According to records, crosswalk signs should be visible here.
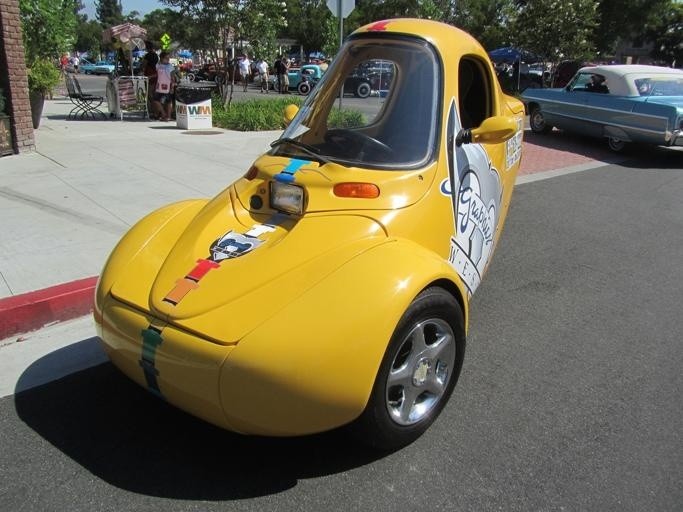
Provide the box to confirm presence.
[160,32,171,45]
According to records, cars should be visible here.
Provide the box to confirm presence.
[498,62,683,156]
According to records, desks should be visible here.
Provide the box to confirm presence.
[107,75,150,120]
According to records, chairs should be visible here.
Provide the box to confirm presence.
[64,74,108,120]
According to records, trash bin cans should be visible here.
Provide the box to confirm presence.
[175,85,213,129]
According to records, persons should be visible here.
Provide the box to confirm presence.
[60,40,291,122]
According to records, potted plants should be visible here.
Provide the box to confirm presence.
[0,86,15,156]
[26,55,61,129]
